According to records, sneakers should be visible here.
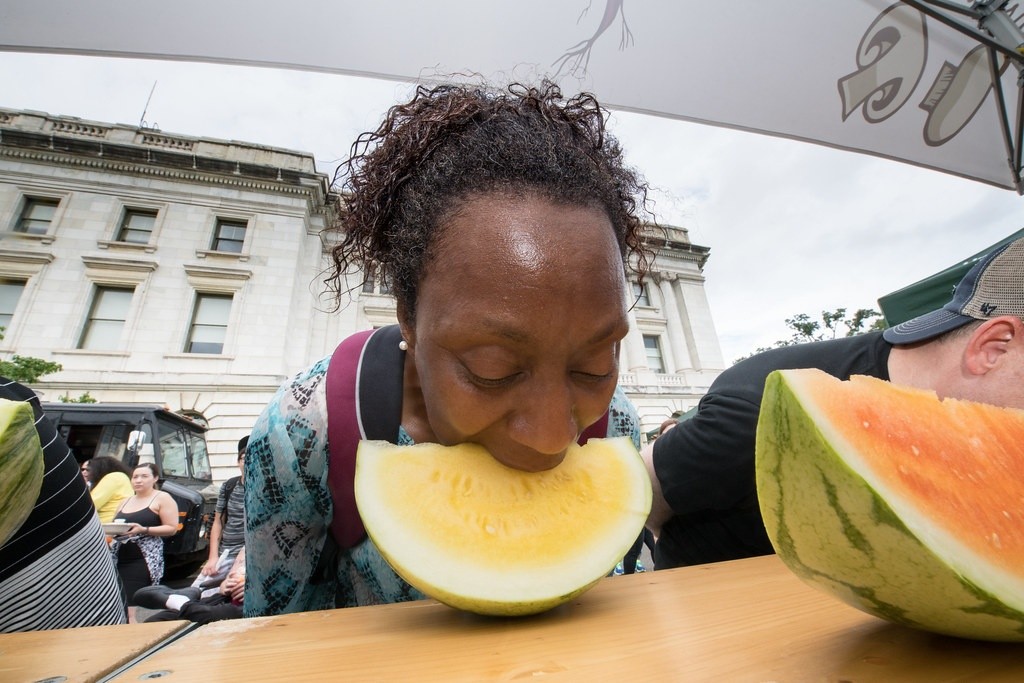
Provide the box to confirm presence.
[614,562,624,575]
[635,559,647,572]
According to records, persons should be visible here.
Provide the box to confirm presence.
[609,237,1024,575]
[0,376,250,635]
[244,71,666,617]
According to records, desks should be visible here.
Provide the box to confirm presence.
[0,553,1024,683]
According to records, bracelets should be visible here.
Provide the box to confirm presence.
[143,526,150,535]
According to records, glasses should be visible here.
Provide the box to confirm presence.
[81,468,88,471]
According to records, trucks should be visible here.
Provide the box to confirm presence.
[40,400,224,583]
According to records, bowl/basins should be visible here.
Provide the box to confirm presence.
[102,523,132,535]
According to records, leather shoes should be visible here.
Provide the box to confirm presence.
[133,585,201,609]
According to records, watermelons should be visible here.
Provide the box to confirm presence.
[353,435,654,614]
[0,397,43,547]
[755,367,1024,642]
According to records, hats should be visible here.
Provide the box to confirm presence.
[238,435,250,462]
[883,228,1024,345]
